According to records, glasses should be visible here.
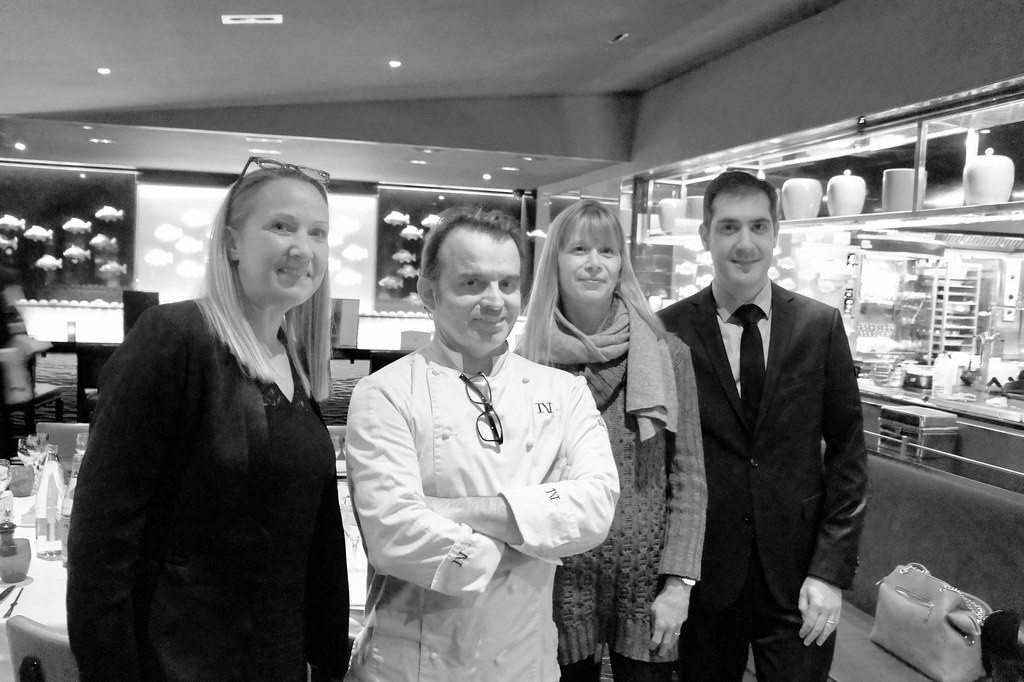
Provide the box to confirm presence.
[459,371,503,445]
[233,157,330,196]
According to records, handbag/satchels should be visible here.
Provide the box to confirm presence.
[869,563,992,682]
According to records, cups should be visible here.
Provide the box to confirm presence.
[7,465,35,497]
[0,459,12,491]
[0,538,31,583]
[0,492,14,523]
[16,433,48,465]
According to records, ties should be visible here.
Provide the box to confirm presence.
[734,304,766,402]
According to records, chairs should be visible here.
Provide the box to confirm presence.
[36,422,89,458]
[6,615,80,682]
[6,354,63,457]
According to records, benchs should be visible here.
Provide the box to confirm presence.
[745,448,1024,682]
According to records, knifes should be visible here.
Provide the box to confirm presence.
[0,586,15,600]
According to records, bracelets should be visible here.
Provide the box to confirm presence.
[678,577,697,586]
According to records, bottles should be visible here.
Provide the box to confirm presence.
[35,442,66,562]
[61,432,89,567]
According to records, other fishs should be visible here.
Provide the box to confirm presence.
[767,242,816,291]
[526,229,547,240]
[327,210,444,305]
[0,205,213,279]
[645,287,668,298]
[675,240,713,301]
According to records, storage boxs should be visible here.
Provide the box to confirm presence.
[400,331,431,350]
[878,404,957,459]
[331,298,360,350]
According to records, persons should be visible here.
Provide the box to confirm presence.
[655,171,870,682]
[342,208,621,681]
[68,170,350,681]
[514,199,707,682]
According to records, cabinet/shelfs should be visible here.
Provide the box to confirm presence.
[628,81,1024,431]
